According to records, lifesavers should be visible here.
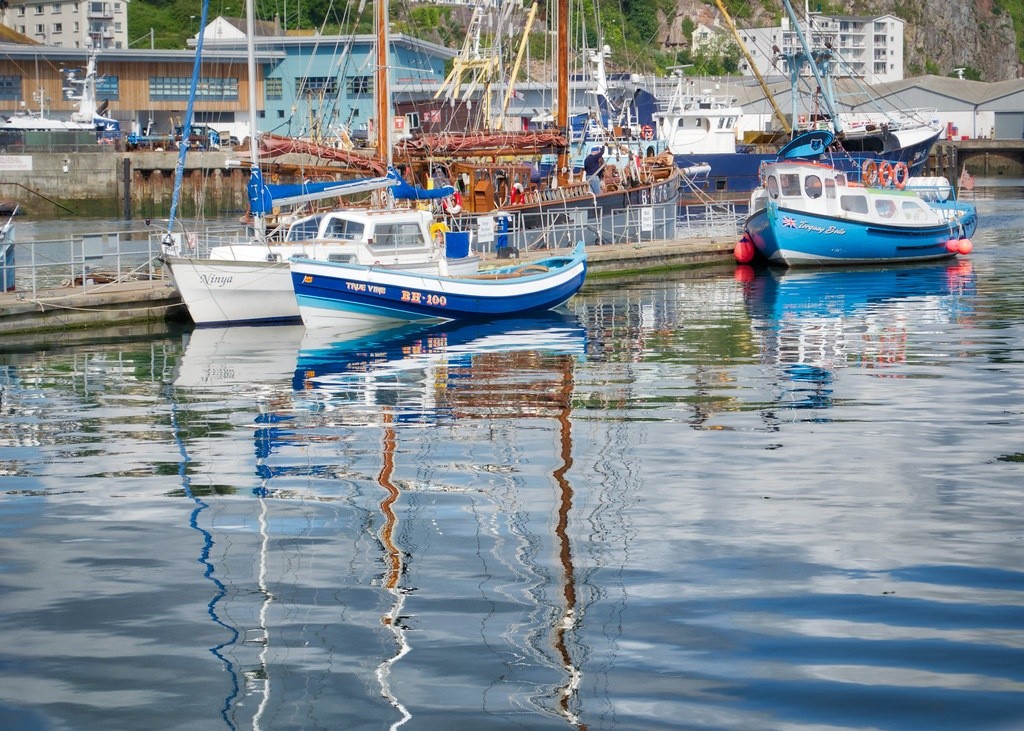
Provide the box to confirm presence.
[511,182,525,207]
[893,162,908,189]
[878,160,893,187]
[441,184,463,215]
[861,158,878,186]
[641,125,653,139]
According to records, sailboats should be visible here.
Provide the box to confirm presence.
[742,259,980,369]
[171,312,591,731]
[741,0,979,268]
[157,1,945,325]
